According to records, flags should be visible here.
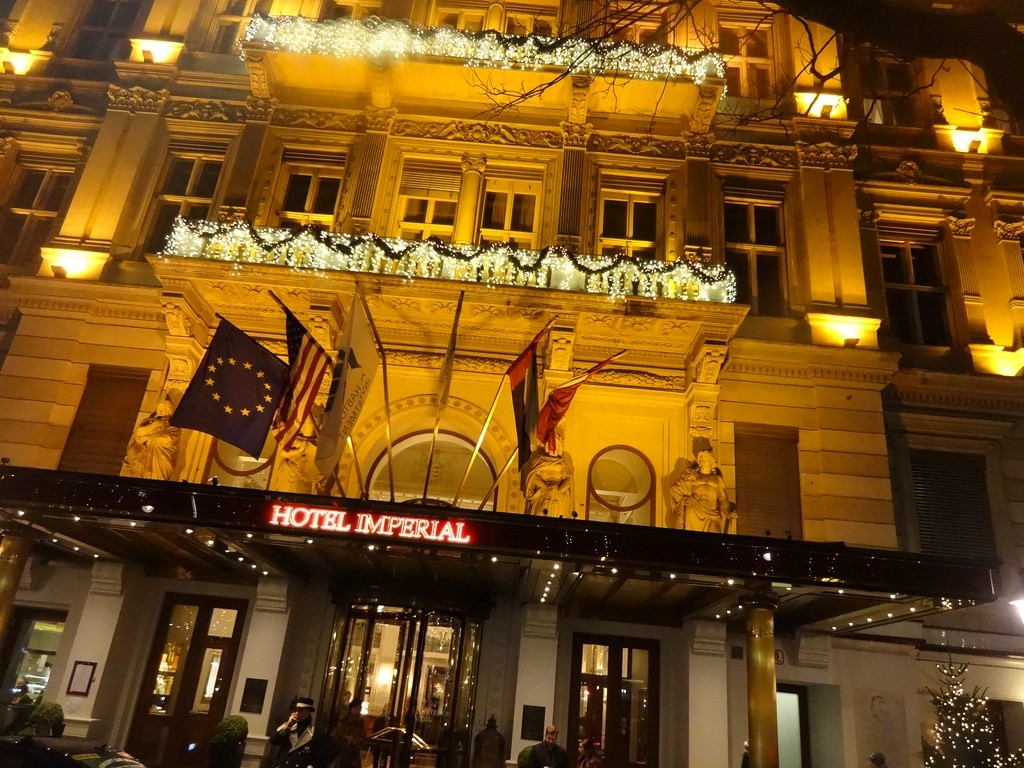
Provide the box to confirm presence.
[509,327,553,467]
[537,357,615,456]
[433,302,465,417]
[169,320,290,460]
[313,294,382,481]
[271,311,333,453]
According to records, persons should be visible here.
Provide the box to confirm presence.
[868,752,888,768]
[742,739,748,768]
[270,697,328,768]
[576,739,605,768]
[671,452,738,533]
[372,703,398,768]
[345,691,351,704]
[331,700,367,768]
[16,675,28,695]
[126,399,178,479]
[527,725,567,768]
[526,437,574,517]
[271,409,322,491]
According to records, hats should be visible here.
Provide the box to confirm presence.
[288,696,315,712]
